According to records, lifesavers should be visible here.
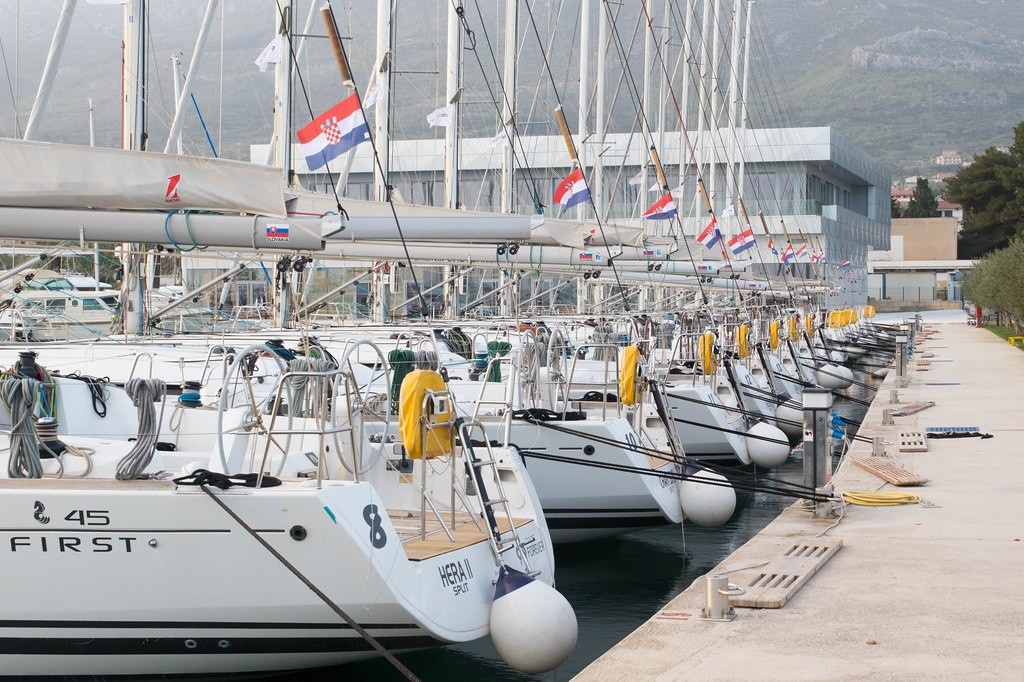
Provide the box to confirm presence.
[770,322,781,349]
[806,316,816,337]
[736,324,753,357]
[828,309,846,328]
[701,332,717,377]
[397,368,455,454]
[618,344,646,405]
[864,304,874,317]
[788,316,798,342]
[844,309,858,325]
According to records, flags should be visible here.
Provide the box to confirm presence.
[295,92,376,173]
[836,257,851,269]
[695,217,723,251]
[640,192,679,220]
[767,237,778,256]
[553,168,592,214]
[727,228,756,256]
[780,244,794,265]
[795,243,828,265]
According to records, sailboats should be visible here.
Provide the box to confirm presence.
[0,0,907,675]
